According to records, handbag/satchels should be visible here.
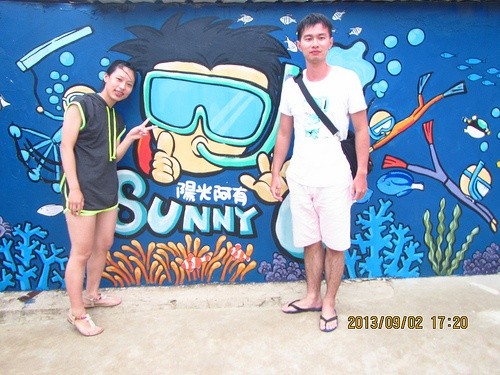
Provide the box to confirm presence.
[340,129,373,181]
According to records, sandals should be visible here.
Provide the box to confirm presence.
[67,309,104,336]
[83,293,121,308]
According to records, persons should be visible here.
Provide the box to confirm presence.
[270,14,370,332]
[59,59,159,337]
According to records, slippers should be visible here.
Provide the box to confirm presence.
[319,308,338,332]
[282,300,322,313]
